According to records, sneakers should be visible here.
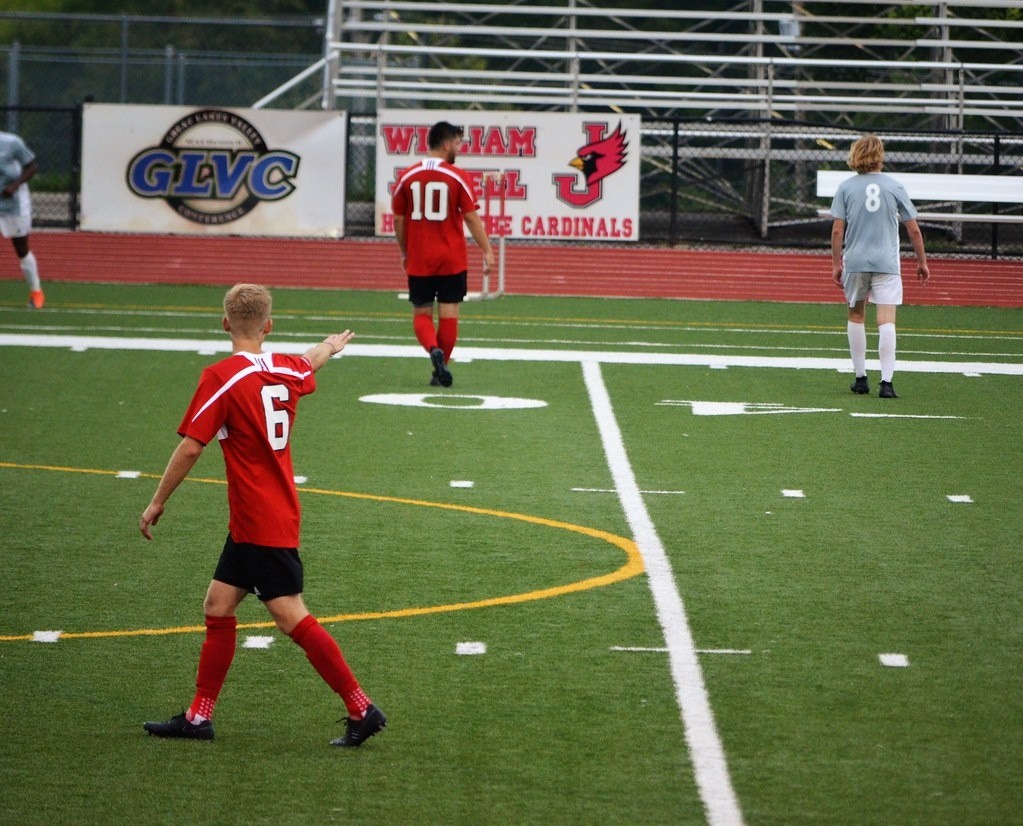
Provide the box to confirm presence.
[330,704,387,748]
[430,377,441,386]
[430,348,452,387]
[29,291,46,310]
[878,381,899,398]
[850,376,869,394]
[143,713,214,740]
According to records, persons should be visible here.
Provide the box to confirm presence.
[139,282,387,749]
[392,121,495,387]
[829,137,931,398]
[0,128,45,313]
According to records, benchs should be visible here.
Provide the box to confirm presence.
[815,170,1023,226]
